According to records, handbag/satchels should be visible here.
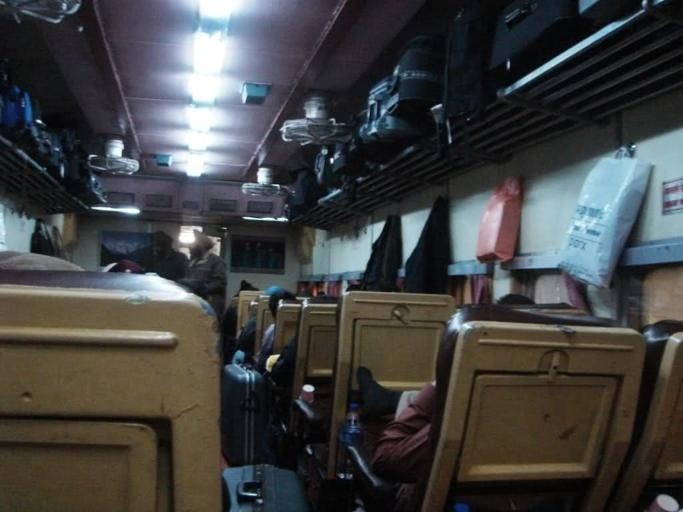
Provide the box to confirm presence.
[555,147,654,294]
[474,170,525,266]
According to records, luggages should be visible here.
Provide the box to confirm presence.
[221,463,310,511]
[291,1,651,212]
[216,359,268,465]
[0,73,113,215]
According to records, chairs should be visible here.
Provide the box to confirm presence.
[0,269,220,511]
[233,286,682,512]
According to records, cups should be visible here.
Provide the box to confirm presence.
[645,494,679,512]
[300,384,315,403]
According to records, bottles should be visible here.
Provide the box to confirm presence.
[336,403,363,479]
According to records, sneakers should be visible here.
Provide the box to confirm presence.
[356,366,394,415]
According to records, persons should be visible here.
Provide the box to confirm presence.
[129,230,187,278]
[221,279,361,424]
[184,229,226,315]
[359,293,535,486]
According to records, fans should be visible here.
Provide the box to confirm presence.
[242,96,354,197]
[87,139,139,176]
